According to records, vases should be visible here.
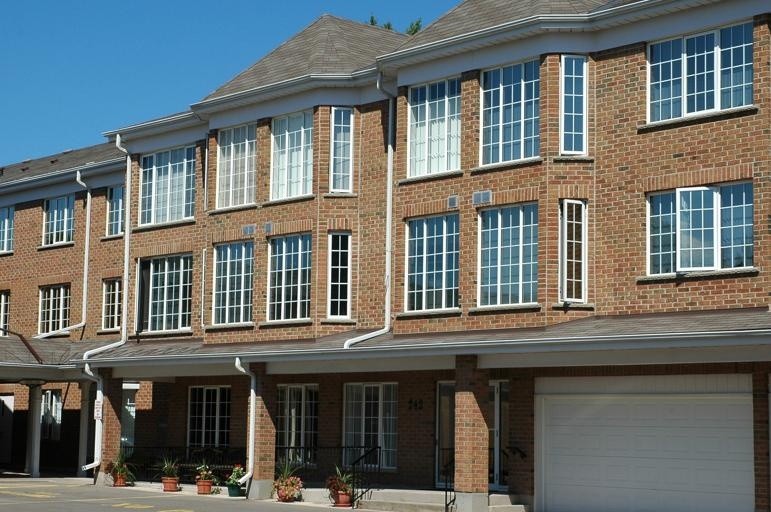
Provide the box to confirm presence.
[228,484,243,497]
[197,480,214,494]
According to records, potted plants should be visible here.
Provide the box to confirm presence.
[326,463,356,507]
[111,452,137,486]
[151,451,181,491]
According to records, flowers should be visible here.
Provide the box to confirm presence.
[271,455,306,503]
[226,462,244,491]
[195,457,220,486]
